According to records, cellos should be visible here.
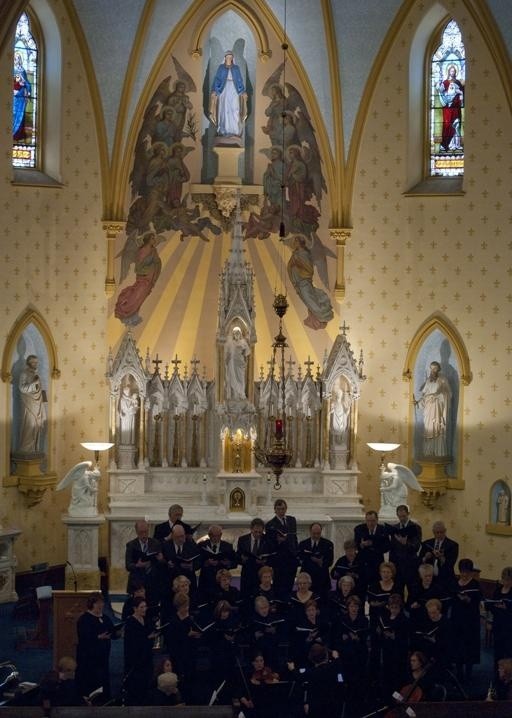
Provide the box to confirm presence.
[386,657,435,717]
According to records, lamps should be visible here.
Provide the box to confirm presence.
[262,0,291,488]
[366,442,401,463]
[81,442,115,466]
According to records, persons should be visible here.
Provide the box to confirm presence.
[209,49,248,137]
[12,50,34,148]
[330,380,353,445]
[17,352,47,456]
[116,379,141,446]
[126,82,200,239]
[249,77,327,238]
[114,233,162,322]
[287,235,336,329]
[436,64,464,151]
[378,471,410,508]
[221,326,250,399]
[414,360,454,458]
[71,469,102,505]
[53,495,511,717]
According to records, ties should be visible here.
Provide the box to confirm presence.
[314,541,316,551]
[436,540,440,551]
[178,545,181,556]
[282,518,285,527]
[253,539,258,554]
[142,543,146,553]
[371,528,373,536]
[212,545,217,553]
[402,524,404,529]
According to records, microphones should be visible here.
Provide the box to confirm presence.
[65,560,77,591]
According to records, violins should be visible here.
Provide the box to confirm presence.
[254,667,279,682]
